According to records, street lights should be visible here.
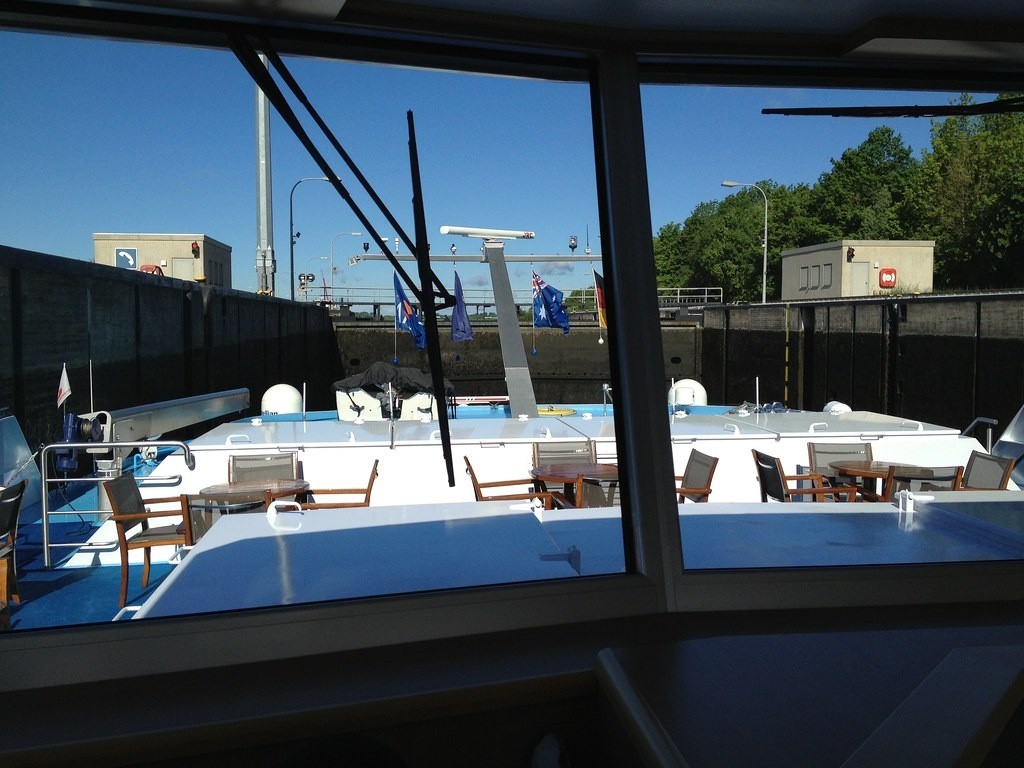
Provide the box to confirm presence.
[290,177,342,301]
[721,181,768,304]
[305,257,327,302]
[332,233,362,302]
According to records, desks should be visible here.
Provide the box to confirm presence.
[530,464,620,509]
[828,460,935,503]
[200,479,311,530]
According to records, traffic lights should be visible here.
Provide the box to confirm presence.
[191,243,199,259]
[846,247,855,263]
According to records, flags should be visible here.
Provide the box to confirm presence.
[532,270,569,336]
[393,270,426,350]
[450,270,475,342]
[56,367,72,408]
[594,270,607,329]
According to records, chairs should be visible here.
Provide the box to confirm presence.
[285,459,379,511]
[176,490,274,556]
[228,453,300,516]
[917,448,1017,492]
[843,466,964,504]
[807,441,876,502]
[534,440,597,507]
[0,479,31,595]
[465,455,552,512]
[102,469,192,608]
[675,449,719,504]
[751,448,857,503]
